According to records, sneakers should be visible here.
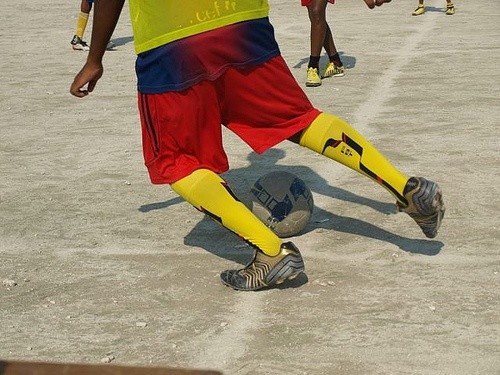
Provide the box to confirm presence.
[221,242,305,290]
[106,40,114,49]
[411,5,425,15]
[70,35,90,51]
[305,67,322,86]
[395,176,445,238]
[446,4,455,15]
[320,62,344,78]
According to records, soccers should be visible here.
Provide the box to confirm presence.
[245,170,315,239]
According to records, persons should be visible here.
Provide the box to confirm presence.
[70,0,445,291]
[300,0,344,86]
[412,0,456,16]
[71,0,116,51]
